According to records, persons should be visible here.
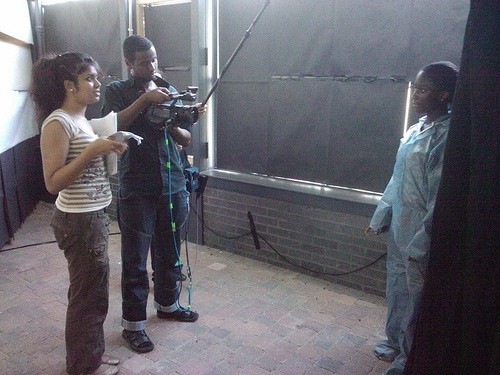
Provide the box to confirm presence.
[366,62,459,375]
[101,34,208,352]
[29,51,129,375]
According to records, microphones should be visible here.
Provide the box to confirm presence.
[170,92,197,102]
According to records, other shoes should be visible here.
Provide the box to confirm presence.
[100,354,120,365]
[90,363,120,375]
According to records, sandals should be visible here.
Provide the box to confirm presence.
[122,327,154,353]
[157,305,199,322]
